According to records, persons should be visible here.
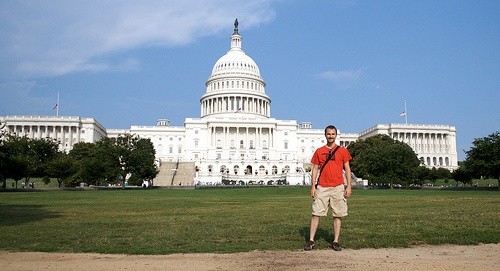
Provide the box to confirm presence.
[304,125,352,251]
[21,181,25,188]
[29,182,32,188]
[179,182,181,185]
[143,184,146,189]
[32,182,35,188]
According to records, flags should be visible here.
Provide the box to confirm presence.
[400,111,405,116]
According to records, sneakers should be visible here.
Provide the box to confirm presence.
[331,242,342,251]
[304,241,315,251]
